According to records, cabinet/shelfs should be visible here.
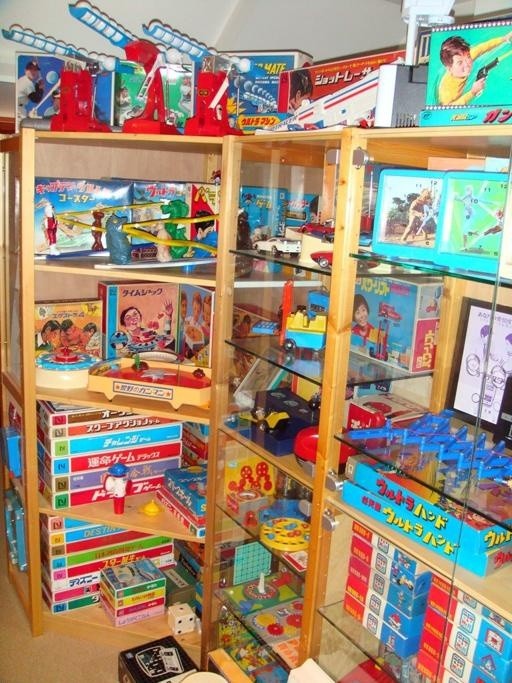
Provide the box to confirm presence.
[0,123,512,683]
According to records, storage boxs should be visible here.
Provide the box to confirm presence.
[15,45,407,133]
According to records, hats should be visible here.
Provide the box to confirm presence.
[25,61,40,72]
